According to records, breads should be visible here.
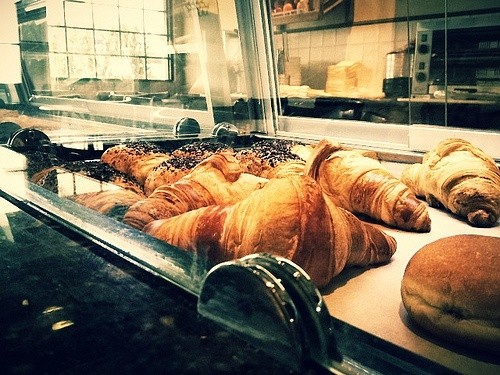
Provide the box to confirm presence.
[269,0,310,15]
[63,135,500,292]
[400,233,500,345]
